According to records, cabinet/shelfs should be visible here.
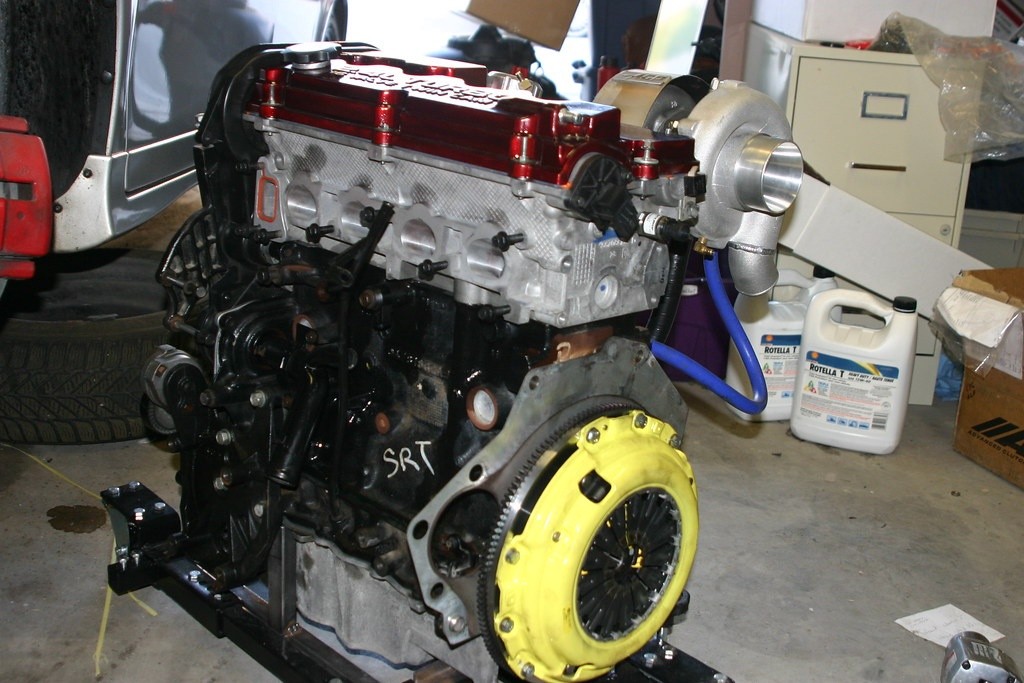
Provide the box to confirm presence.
[740,23,990,404]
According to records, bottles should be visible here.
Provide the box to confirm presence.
[788,288,918,454]
[724,265,842,421]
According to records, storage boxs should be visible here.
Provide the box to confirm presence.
[749,0,997,44]
[930,267,1024,490]
